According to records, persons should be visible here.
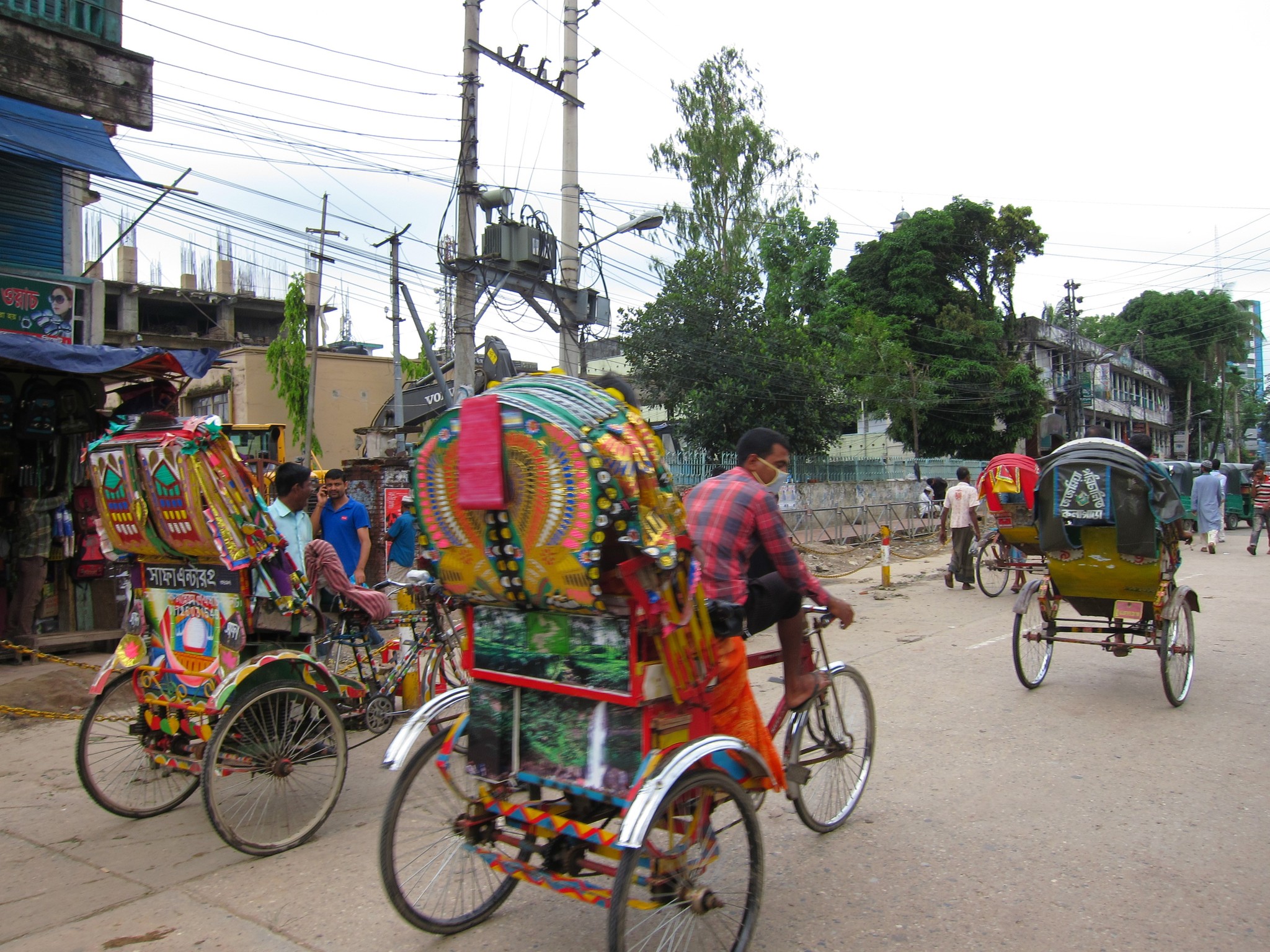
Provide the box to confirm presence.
[1210,458,1227,543]
[683,426,855,712]
[310,468,398,669]
[1113,434,1194,657]
[1257,460,1265,471]
[926,476,948,506]
[939,467,981,590]
[383,496,417,592]
[1190,460,1222,554]
[1010,544,1027,591]
[1085,425,1112,439]
[252,462,314,607]
[918,486,941,519]
[1247,463,1270,555]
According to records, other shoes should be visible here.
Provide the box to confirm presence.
[1262,526,1268,529]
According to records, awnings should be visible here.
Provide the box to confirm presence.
[1,334,237,413]
[0,97,151,188]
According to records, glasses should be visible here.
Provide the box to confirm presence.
[29,308,72,336]
[47,295,70,304]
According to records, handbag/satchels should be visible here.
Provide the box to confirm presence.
[689,597,752,640]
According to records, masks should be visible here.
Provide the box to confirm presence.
[750,457,790,495]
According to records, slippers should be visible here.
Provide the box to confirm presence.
[963,583,975,590]
[302,742,339,763]
[1218,540,1224,542]
[1267,551,1270,554]
[944,575,953,588]
[1011,585,1022,592]
[1208,545,1215,554]
[792,670,833,713]
[374,624,396,631]
[1247,547,1256,555]
[1201,548,1207,552]
[1113,644,1128,657]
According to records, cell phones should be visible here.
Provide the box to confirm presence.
[323,487,328,496]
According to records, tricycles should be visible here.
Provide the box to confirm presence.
[78,424,465,856]
[975,456,1050,597]
[379,373,877,952]
[1160,458,1256,533]
[1011,439,1200,706]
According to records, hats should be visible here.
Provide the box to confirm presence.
[401,496,414,503]
[925,486,932,491]
[1201,460,1213,468]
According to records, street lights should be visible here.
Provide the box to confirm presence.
[561,211,667,374]
